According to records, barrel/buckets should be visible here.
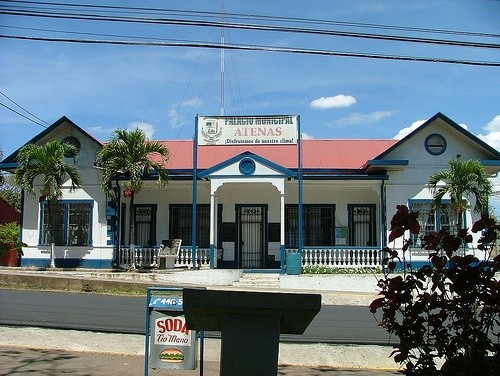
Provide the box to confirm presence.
[286,252,302,275]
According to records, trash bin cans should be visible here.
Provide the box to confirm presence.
[285,249,302,275]
